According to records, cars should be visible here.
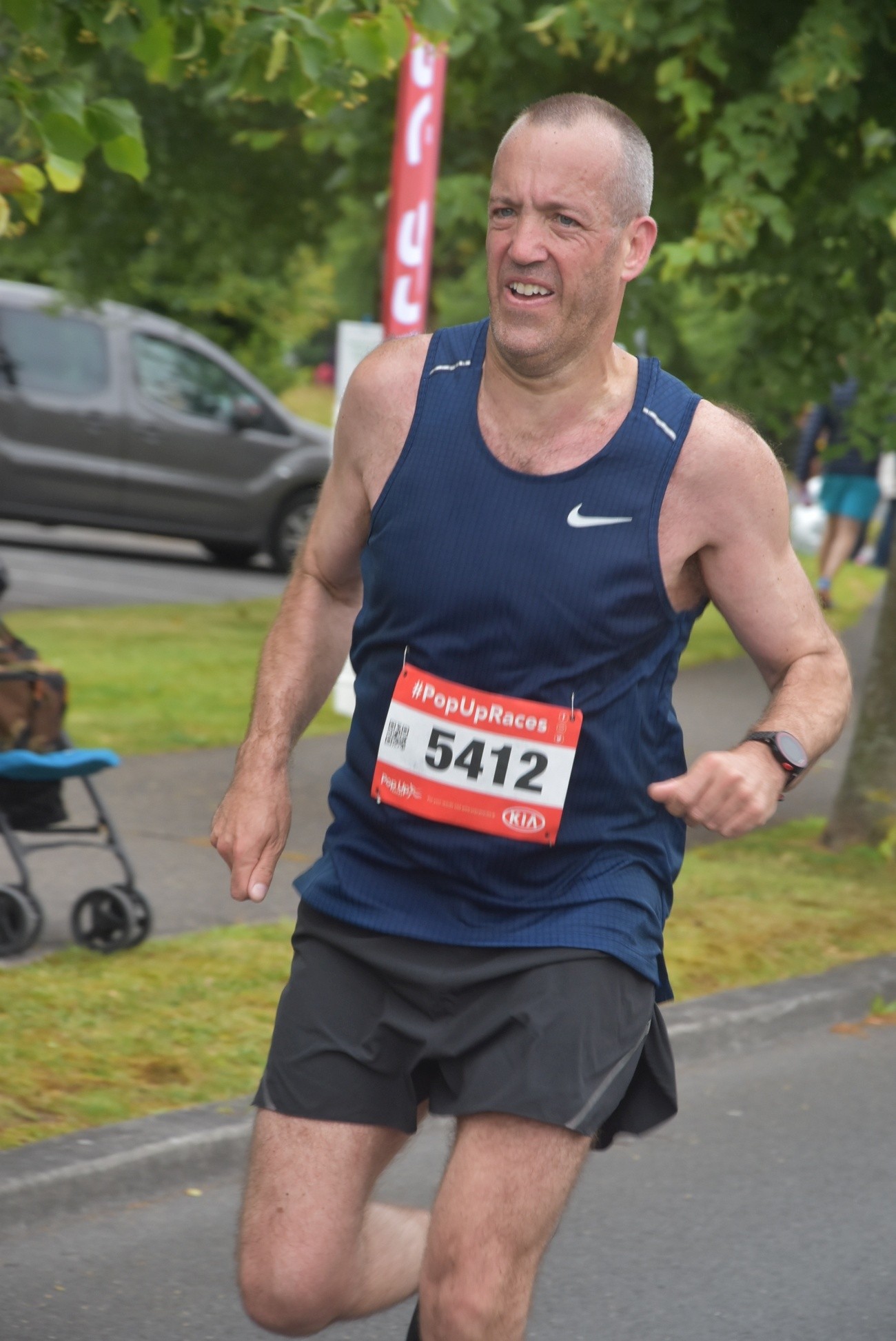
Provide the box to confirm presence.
[0,279,331,572]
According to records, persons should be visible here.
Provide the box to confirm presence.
[210,91,854,1341]
[794,375,896,611]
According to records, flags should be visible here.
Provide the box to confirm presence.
[382,16,446,338]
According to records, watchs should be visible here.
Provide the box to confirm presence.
[740,731,809,802]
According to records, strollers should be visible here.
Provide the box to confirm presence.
[0,565,153,955]
